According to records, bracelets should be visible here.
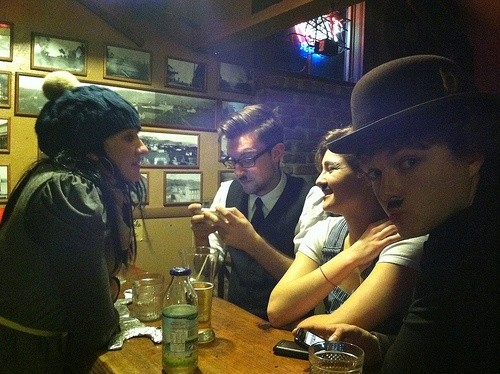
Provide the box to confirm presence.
[319,266,338,288]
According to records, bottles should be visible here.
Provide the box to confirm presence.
[160,267,199,374]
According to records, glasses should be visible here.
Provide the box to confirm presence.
[218,143,274,169]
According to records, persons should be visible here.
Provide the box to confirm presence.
[268,55,500,374]
[187,104,330,333]
[1,71,148,374]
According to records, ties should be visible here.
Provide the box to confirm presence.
[251,197,265,233]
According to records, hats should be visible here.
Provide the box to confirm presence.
[33,71,141,156]
[326,55,481,155]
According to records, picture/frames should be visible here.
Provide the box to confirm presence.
[163,171,203,206]
[0,163,11,203]
[131,171,149,205]
[217,133,232,162]
[103,42,153,85]
[30,31,89,77]
[0,70,12,109]
[14,72,219,132]
[217,59,255,96]
[0,116,11,153]
[0,20,14,62]
[219,98,257,125]
[37,129,200,169]
[163,55,208,93]
[217,170,237,192]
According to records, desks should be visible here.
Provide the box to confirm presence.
[90,275,348,374]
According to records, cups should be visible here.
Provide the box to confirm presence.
[130,271,165,323]
[180,246,220,344]
[308,341,365,374]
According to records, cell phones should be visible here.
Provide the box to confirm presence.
[273,339,309,360]
[294,328,327,352]
[194,208,227,223]
